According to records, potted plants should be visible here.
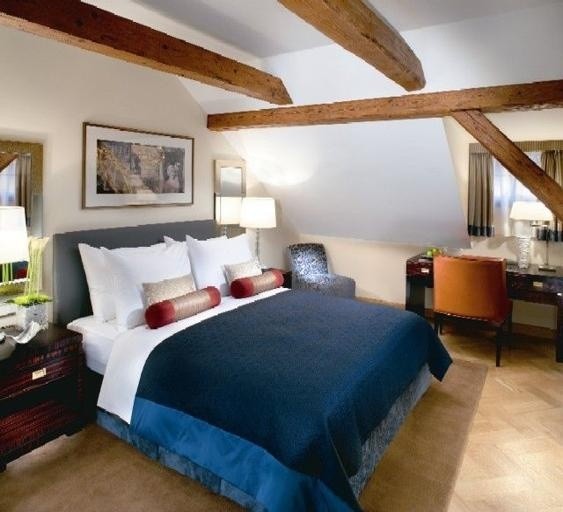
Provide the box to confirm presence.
[4,292,54,332]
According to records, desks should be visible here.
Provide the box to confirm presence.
[403,250,562,363]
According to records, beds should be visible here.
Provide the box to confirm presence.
[48,218,454,512]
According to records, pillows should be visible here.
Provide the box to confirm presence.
[99,240,197,330]
[75,242,166,323]
[229,267,284,299]
[161,232,227,254]
[140,273,195,309]
[221,257,263,296]
[183,232,264,298]
[143,285,221,329]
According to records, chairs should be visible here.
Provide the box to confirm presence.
[285,242,356,298]
[430,252,514,368]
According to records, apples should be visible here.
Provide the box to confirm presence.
[427,251,433,256]
[433,248,437,252]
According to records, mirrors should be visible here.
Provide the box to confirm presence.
[214,158,246,238]
[0,133,44,299]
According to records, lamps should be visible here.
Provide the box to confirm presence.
[0,205,32,265]
[509,200,557,272]
[237,196,277,261]
[215,195,243,235]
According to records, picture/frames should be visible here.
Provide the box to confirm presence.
[80,120,194,209]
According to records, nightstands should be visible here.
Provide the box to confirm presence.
[0,320,86,473]
[260,267,292,290]
